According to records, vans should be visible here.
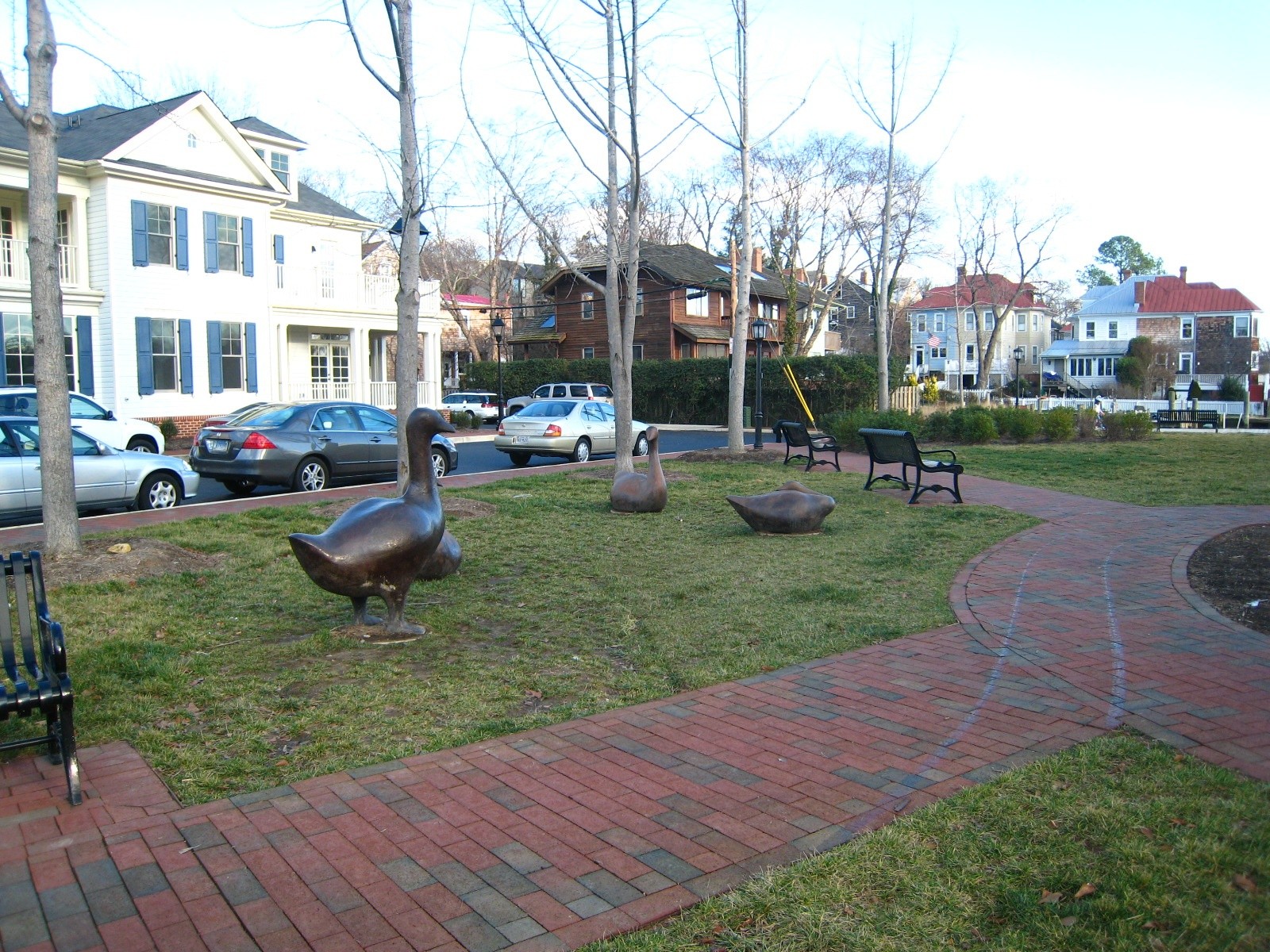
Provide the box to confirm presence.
[441,391,505,425]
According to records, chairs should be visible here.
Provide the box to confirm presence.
[16,398,30,417]
[0,433,26,456]
[333,416,350,430]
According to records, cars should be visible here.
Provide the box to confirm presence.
[0,414,201,529]
[186,401,459,495]
[0,384,167,457]
[494,396,650,466]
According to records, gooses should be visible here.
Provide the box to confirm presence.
[289,408,462,639]
[610,426,668,513]
[725,480,836,535]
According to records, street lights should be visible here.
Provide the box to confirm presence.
[751,316,768,451]
[1013,348,1024,408]
[490,313,505,431]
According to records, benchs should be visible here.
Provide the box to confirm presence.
[858,428,964,503]
[782,421,842,473]
[0,551,83,805]
[1156,410,1220,433]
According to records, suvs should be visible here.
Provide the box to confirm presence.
[503,382,614,417]
